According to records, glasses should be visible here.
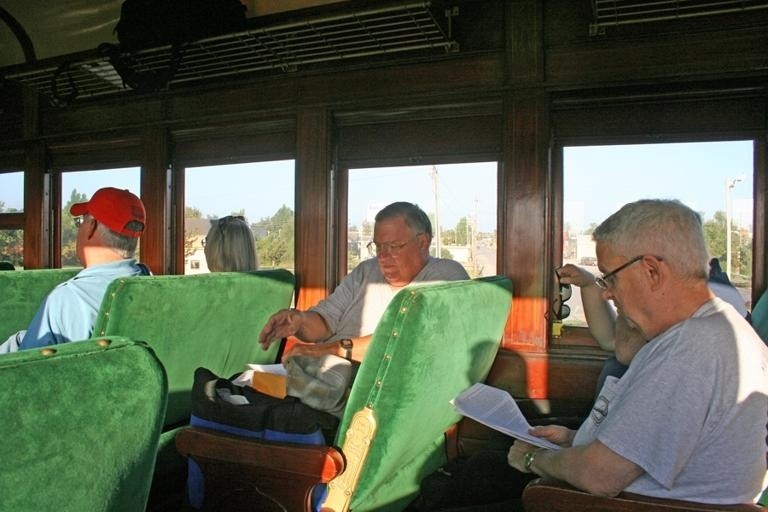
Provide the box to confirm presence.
[595,255,662,290]
[219,216,244,233]
[552,267,571,319]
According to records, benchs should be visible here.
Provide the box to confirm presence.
[515,285,768,511]
[1,266,88,347]
[152,272,517,511]
[0,333,171,512]
[86,264,300,431]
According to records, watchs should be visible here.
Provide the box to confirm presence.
[340,338,354,360]
[524,447,542,472]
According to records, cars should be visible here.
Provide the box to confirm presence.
[578,256,597,266]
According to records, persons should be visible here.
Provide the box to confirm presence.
[258,202,470,397]
[0,186,154,359]
[506,199,768,508]
[528,214,751,431]
[201,214,257,274]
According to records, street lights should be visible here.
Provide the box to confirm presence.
[430,165,442,258]
[724,173,744,280]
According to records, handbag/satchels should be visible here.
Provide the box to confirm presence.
[187,366,341,511]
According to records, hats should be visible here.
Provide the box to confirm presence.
[71,188,146,237]
